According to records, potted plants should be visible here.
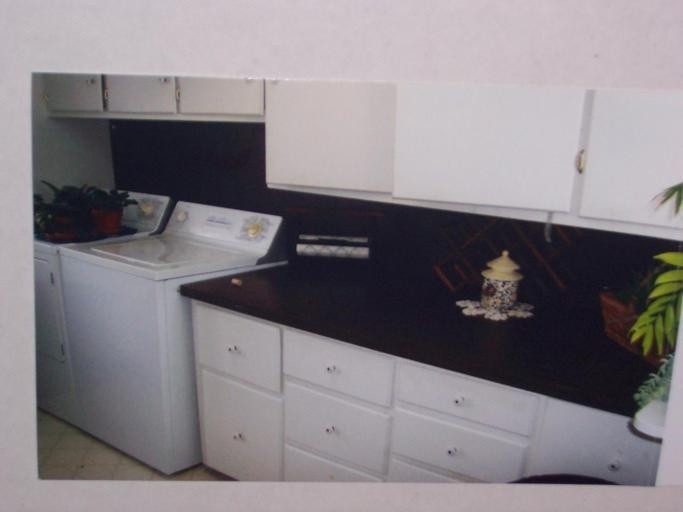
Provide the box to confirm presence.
[34,177,139,243]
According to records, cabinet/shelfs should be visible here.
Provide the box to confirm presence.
[391,356,540,484]
[192,297,284,483]
[541,394,660,487]
[392,81,585,231]
[44,74,106,117]
[104,75,179,122]
[264,79,394,205]
[177,77,266,124]
[282,327,391,484]
[585,87,683,242]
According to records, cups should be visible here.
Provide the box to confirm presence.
[482,249,524,312]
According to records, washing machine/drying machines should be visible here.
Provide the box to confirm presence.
[57,198,284,478]
[31,188,176,434]
